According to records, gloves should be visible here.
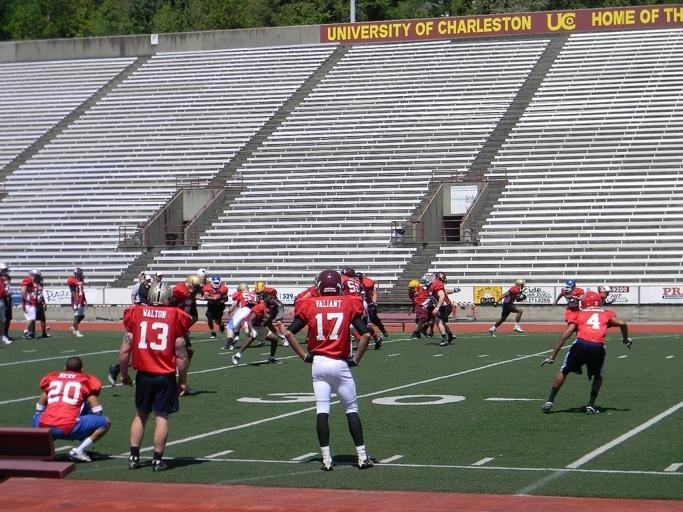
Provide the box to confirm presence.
[347,357,359,367]
[303,353,314,363]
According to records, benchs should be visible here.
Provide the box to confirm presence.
[0,428,75,479]
[0,55,138,170]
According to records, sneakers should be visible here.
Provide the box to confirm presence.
[152,460,168,471]
[357,457,374,469]
[586,406,599,413]
[231,355,240,365]
[320,461,335,470]
[129,456,141,469]
[383,330,388,337]
[439,340,447,345]
[278,338,290,346]
[375,336,383,350]
[72,330,84,337]
[24,330,35,339]
[69,448,91,463]
[224,336,240,351]
[268,357,279,365]
[108,363,119,386]
[220,324,225,333]
[42,334,49,338]
[2,336,11,344]
[514,326,525,332]
[68,327,77,333]
[209,336,216,339]
[488,328,496,338]
[541,402,553,410]
[448,338,455,345]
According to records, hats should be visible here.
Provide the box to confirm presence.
[138,272,145,277]
[146,274,151,280]
[156,272,162,276]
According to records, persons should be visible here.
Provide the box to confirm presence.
[220,283,258,350]
[190,269,206,329]
[284,271,373,471]
[108,276,201,386]
[489,280,526,338]
[232,287,284,366]
[0,263,12,345]
[67,268,88,338]
[539,291,633,414]
[554,279,584,324]
[2,274,13,339]
[339,267,388,350]
[120,282,189,471]
[131,272,163,305]
[283,275,320,347]
[33,357,111,462]
[33,277,51,338]
[203,276,228,338]
[406,273,460,346]
[599,287,617,305]
[22,270,41,340]
[250,282,285,338]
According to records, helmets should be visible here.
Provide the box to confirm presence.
[73,268,82,274]
[29,269,41,275]
[148,281,172,306]
[581,291,602,310]
[409,280,420,287]
[197,269,206,277]
[318,270,341,295]
[237,283,248,291]
[599,286,610,292]
[515,280,526,285]
[187,274,203,289]
[435,272,447,283]
[0,263,9,269]
[211,275,221,289]
[564,279,576,292]
[342,267,355,276]
[422,273,437,286]
[255,281,266,292]
